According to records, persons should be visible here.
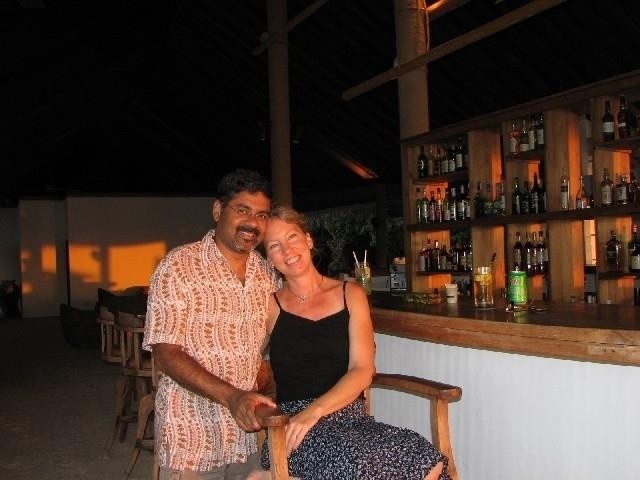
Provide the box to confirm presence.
[259,206,451,480]
[140,165,283,480]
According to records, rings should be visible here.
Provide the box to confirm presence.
[292,435,295,436]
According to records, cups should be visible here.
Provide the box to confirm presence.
[446,287,458,304]
[354,262,371,295]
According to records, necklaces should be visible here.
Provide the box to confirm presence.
[288,275,323,304]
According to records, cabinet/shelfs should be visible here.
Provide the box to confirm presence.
[400,71,640,306]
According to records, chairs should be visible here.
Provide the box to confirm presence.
[247,372,462,480]
[96,317,134,457]
[113,314,159,480]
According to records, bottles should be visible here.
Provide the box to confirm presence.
[615,174,631,206]
[466,182,472,220]
[458,184,466,221]
[421,191,430,224]
[600,168,613,206]
[416,188,421,223]
[442,150,449,174]
[524,232,533,277]
[429,191,436,224]
[449,143,457,173]
[465,140,470,170]
[532,232,540,274]
[417,146,427,178]
[472,266,493,307]
[457,138,464,170]
[540,230,549,273]
[601,100,615,142]
[530,118,537,151]
[416,239,471,271]
[559,167,569,211]
[519,120,530,152]
[628,224,640,272]
[604,230,624,272]
[427,145,434,177]
[537,114,545,150]
[513,231,524,271]
[474,181,507,218]
[617,96,631,138]
[512,173,547,214]
[451,188,458,222]
[629,179,640,203]
[444,191,451,222]
[436,189,444,224]
[509,124,519,156]
[435,149,441,177]
[576,176,589,209]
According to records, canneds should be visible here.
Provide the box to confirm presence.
[507,266,527,308]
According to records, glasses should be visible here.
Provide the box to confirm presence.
[222,201,269,222]
